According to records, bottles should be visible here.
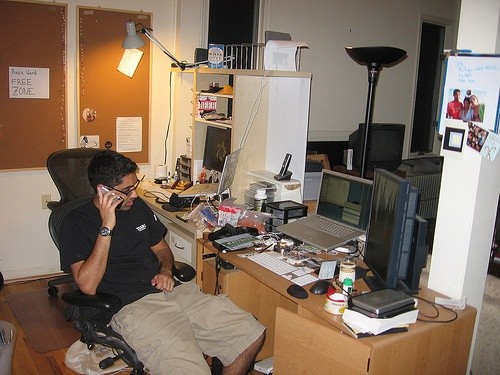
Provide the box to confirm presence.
[167,176,171,183]
[342,278,352,295]
[339,261,356,283]
[254,189,267,212]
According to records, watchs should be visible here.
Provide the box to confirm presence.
[98,226,113,236]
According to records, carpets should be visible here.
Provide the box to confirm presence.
[7,286,79,354]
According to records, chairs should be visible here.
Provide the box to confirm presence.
[47,148,223,374]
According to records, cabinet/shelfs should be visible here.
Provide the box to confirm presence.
[131,67,476,375]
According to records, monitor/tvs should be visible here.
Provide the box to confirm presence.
[349,123,405,173]
[360,168,428,296]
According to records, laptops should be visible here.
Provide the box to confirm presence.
[178,148,241,197]
[276,168,374,251]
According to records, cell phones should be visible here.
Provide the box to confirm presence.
[99,186,124,209]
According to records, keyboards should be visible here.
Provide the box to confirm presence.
[247,251,319,286]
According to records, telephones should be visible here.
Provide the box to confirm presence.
[274,153,293,180]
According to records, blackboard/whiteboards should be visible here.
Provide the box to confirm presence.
[436,52,500,144]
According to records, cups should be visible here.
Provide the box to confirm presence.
[174,175,178,180]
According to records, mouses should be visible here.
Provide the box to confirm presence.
[309,280,331,295]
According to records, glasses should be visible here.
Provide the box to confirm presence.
[108,179,140,196]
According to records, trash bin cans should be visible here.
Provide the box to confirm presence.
[0,320,18,375]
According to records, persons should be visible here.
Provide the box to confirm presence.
[447,89,489,152]
[59,150,266,375]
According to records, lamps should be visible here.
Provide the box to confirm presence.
[124,20,188,71]
[345,43,406,179]
